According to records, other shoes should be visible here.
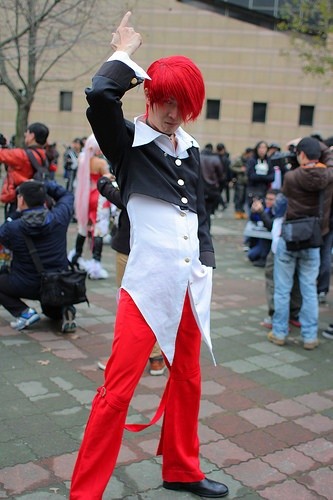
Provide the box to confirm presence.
[265,316,272,327]
[304,339,320,349]
[235,212,242,219]
[242,212,248,218]
[253,259,265,267]
[290,320,300,327]
[318,292,326,303]
[322,330,333,339]
[268,332,284,345]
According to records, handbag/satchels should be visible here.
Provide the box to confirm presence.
[41,271,89,306]
[282,217,321,251]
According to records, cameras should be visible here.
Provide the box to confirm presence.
[272,145,298,172]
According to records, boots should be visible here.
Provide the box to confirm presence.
[72,234,86,263]
[92,237,103,263]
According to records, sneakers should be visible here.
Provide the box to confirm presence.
[98,360,107,370]
[61,306,77,332]
[10,307,39,330]
[150,358,166,375]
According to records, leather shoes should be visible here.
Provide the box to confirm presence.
[162,478,228,497]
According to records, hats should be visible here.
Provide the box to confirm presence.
[268,144,280,151]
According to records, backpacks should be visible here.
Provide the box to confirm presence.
[26,149,50,180]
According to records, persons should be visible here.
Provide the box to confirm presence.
[68,6,230,499]
[0,122,333,352]
